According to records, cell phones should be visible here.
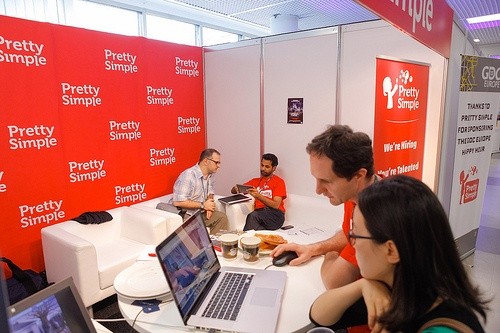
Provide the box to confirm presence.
[281,225,293,229]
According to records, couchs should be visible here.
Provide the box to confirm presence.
[40,190,313,319]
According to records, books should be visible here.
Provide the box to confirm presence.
[218,194,252,206]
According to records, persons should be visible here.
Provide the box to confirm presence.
[271,124,383,290]
[309,173,495,333]
[164,263,200,293]
[172,148,229,236]
[231,153,288,231]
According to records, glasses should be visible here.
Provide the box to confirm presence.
[347,218,375,244]
[207,157,221,165]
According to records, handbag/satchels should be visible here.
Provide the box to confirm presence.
[0,256,55,307]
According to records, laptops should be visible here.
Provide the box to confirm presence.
[156,209,287,333]
[7,275,97,333]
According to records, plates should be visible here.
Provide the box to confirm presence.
[113,261,172,301]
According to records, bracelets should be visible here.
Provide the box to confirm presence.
[200,202,204,209]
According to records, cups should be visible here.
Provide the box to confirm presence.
[240,236,261,264]
[220,233,240,261]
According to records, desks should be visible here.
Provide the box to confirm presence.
[118,230,343,333]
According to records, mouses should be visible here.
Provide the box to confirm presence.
[272,251,297,267]
